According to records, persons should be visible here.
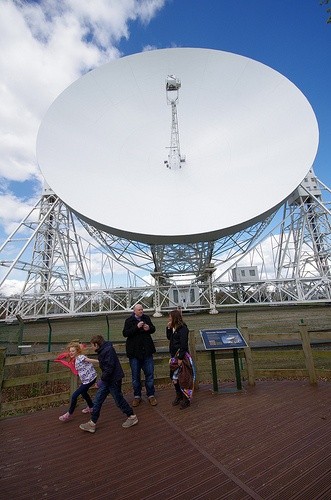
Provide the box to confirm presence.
[166,310,191,409]
[80,335,138,433]
[122,304,158,406]
[58,342,100,422]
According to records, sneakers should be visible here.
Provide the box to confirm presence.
[81,407,93,414]
[59,412,74,421]
[122,415,138,427]
[79,422,96,433]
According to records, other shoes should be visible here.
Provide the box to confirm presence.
[148,397,158,406]
[172,395,182,405]
[179,400,190,409]
[132,398,141,407]
